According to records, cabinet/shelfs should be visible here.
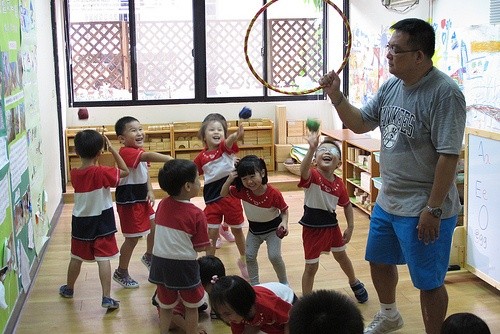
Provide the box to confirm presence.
[320,132,344,181]
[173,119,274,179]
[345,141,380,216]
[65,123,173,186]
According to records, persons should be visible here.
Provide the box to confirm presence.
[113,116,176,288]
[298,130,369,304]
[211,221,236,247]
[441,312,491,334]
[153,254,223,329]
[291,289,364,334]
[210,273,299,334]
[319,19,467,334]
[188,120,249,278]
[220,154,289,286]
[146,159,210,334]
[61,129,130,309]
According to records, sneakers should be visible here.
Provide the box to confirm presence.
[349,278,368,304]
[362,310,404,334]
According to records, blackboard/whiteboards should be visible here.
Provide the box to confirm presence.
[464,127,500,290]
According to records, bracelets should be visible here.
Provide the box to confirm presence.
[330,91,344,107]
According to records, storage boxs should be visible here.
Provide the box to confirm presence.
[276,105,287,143]
[274,144,291,163]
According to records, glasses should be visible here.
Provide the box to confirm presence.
[385,43,418,55]
[316,146,339,154]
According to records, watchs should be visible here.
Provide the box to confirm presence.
[425,205,442,217]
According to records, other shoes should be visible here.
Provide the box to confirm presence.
[101,296,120,309]
[59,284,74,299]
[140,252,153,272]
[112,268,139,289]
